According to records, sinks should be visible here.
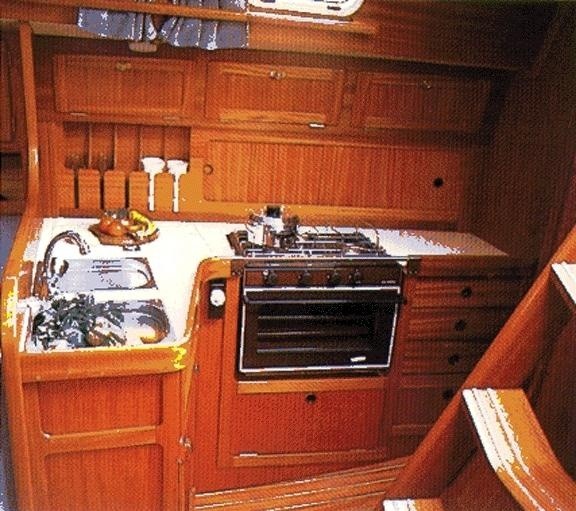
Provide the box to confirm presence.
[30,257,158,293]
[25,295,176,353]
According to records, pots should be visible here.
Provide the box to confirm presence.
[245,205,300,249]
[235,268,402,376]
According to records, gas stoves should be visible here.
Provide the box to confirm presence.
[226,225,410,268]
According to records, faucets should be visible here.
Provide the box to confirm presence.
[41,229,91,294]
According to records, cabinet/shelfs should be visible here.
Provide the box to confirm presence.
[388,275,530,460]
[2,348,186,511]
